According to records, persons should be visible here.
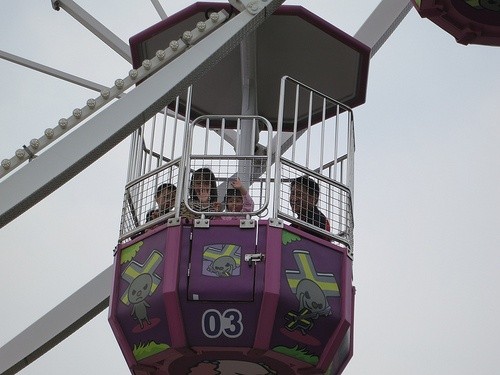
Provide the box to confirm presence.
[176,167,223,220]
[219,177,255,221]
[141,182,176,228]
[286,176,333,244]
